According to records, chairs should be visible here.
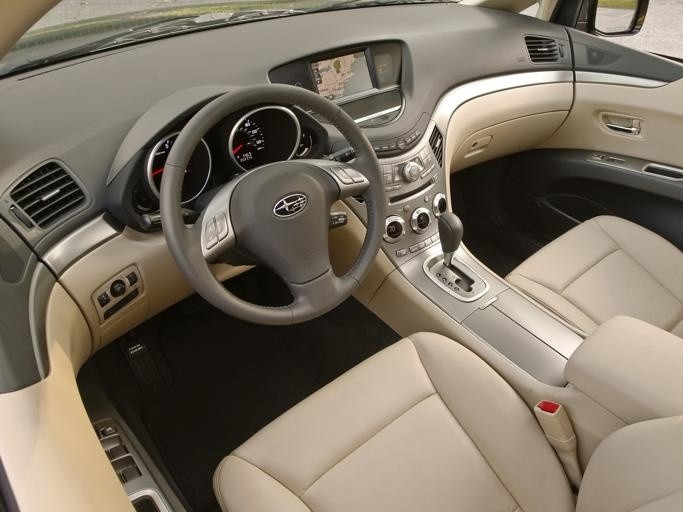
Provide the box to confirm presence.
[213,330,682,511]
[508,213,681,337]
